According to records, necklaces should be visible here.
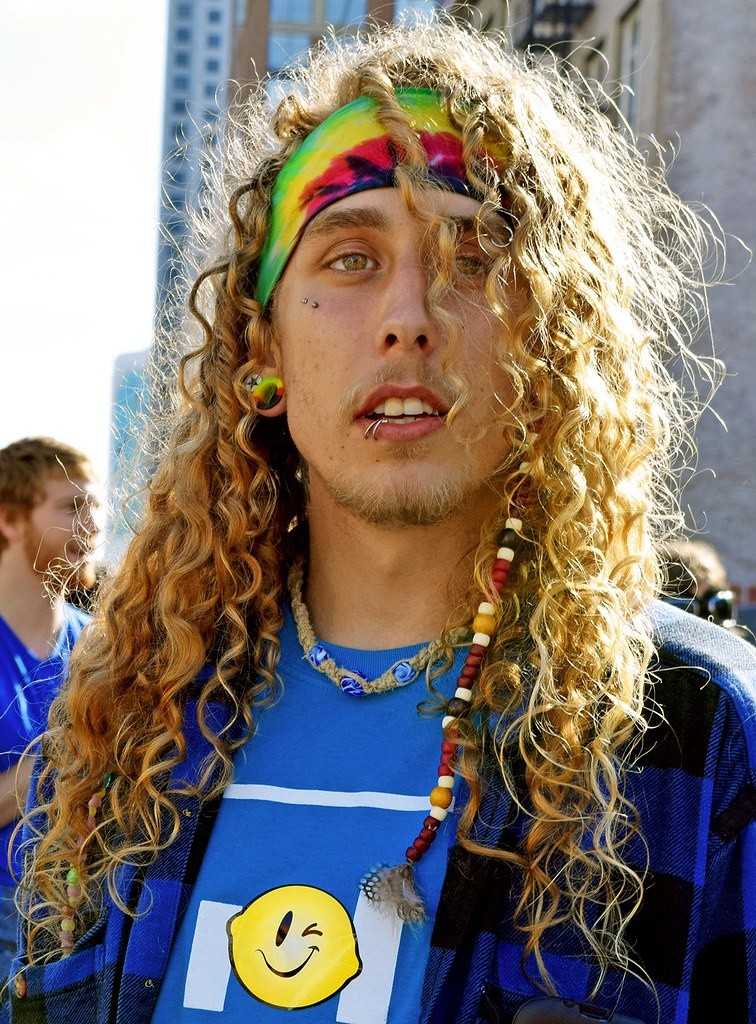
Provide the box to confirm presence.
[287,554,479,699]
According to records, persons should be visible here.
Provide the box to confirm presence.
[0,0,756,1024]
[0,435,103,1024]
[651,537,756,649]
